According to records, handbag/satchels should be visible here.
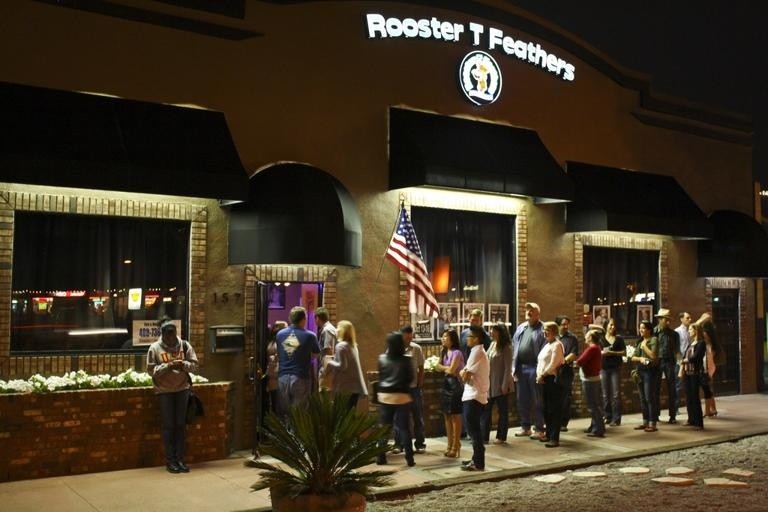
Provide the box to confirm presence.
[185,393,204,425]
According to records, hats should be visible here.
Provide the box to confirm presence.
[525,302,540,314]
[654,309,672,318]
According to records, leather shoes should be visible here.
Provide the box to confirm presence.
[178,462,188,472]
[167,463,178,473]
[584,419,620,437]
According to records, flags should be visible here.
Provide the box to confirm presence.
[382,200,441,321]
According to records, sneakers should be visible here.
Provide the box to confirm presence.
[634,411,718,431]
[376,442,426,465]
[516,427,567,446]
[444,433,503,471]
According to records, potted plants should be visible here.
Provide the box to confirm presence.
[245,374,394,512]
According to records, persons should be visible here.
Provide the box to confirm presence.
[374,323,428,467]
[144,321,199,474]
[572,318,625,437]
[510,302,580,450]
[264,307,368,434]
[429,309,517,472]
[624,307,718,433]
